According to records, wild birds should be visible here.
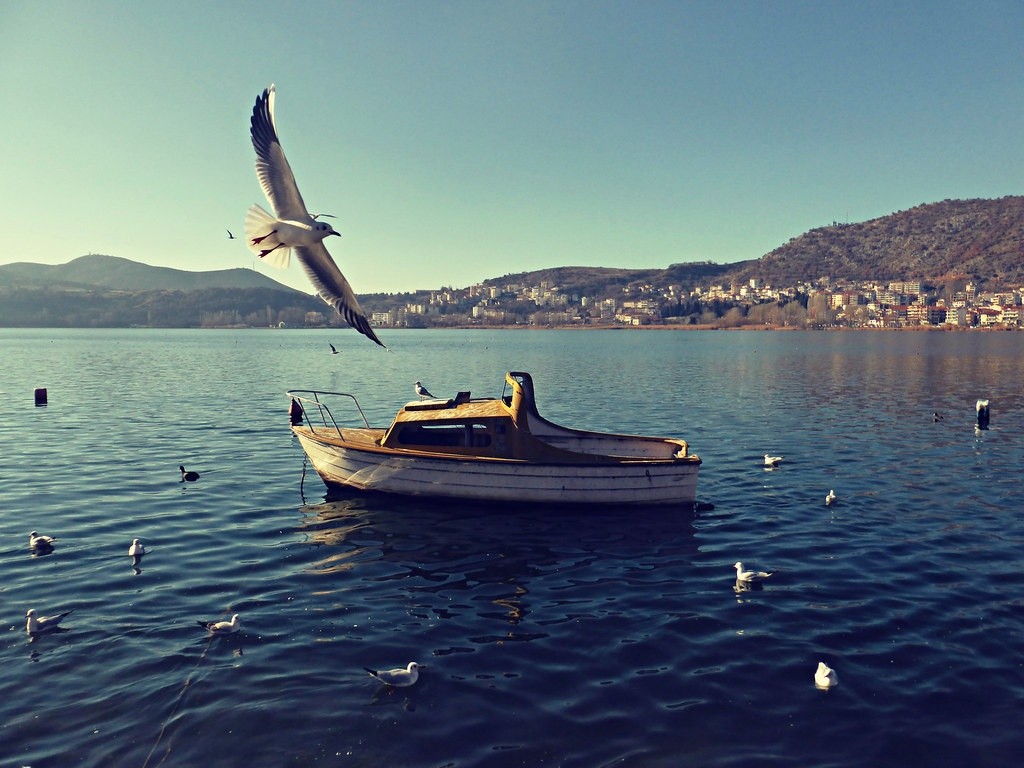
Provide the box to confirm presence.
[764,455,785,465]
[28,532,58,551]
[813,660,841,690]
[196,614,243,636]
[245,80,394,349]
[412,380,441,401]
[24,611,78,633]
[825,489,837,505]
[361,662,427,687]
[129,539,155,558]
[732,561,775,581]
[178,465,201,483]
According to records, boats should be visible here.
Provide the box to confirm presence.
[286,387,703,513]
